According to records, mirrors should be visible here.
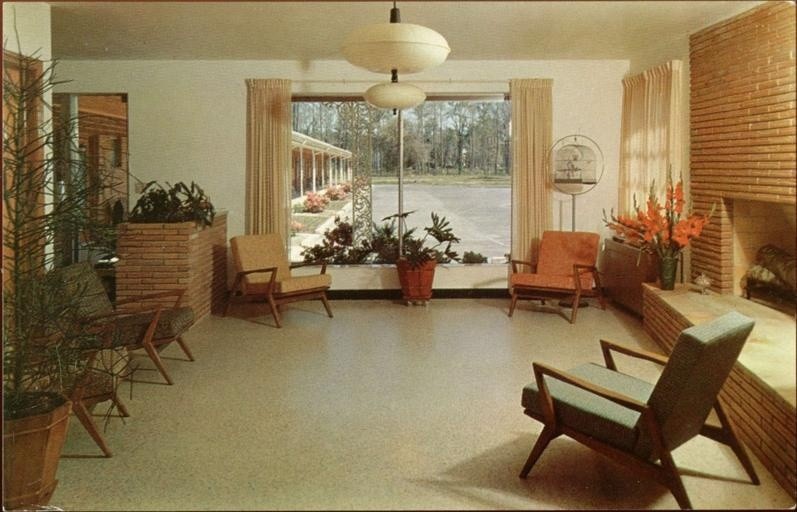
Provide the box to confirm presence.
[53,93,128,268]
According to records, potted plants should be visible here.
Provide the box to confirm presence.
[0,5,157,512]
[372,210,462,305]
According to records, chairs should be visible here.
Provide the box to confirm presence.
[2,336,129,457]
[508,230,607,324]
[221,233,334,327]
[47,261,195,385]
[519,312,760,512]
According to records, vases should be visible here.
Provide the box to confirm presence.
[659,257,678,290]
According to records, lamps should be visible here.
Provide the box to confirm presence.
[340,0,451,115]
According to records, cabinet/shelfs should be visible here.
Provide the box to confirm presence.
[605,239,658,316]
[641,0,796,503]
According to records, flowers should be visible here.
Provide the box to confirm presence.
[602,164,717,267]
